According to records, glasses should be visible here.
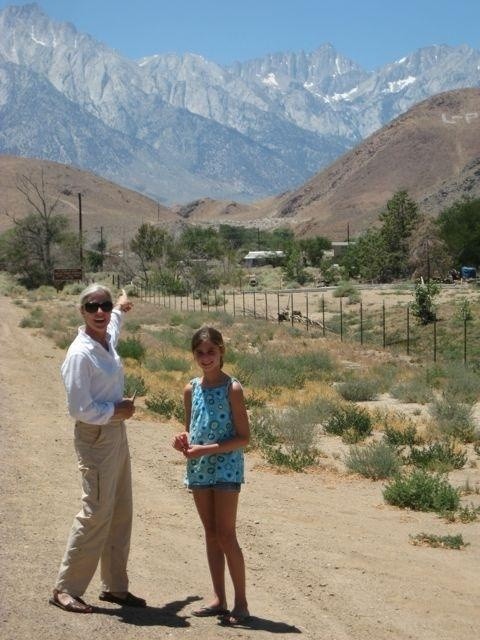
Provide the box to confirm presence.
[82,300,115,314]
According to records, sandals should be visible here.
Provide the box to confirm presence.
[99,588,148,609]
[49,589,97,613]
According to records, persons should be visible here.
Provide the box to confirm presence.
[173,323,253,624]
[50,282,148,614]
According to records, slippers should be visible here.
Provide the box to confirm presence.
[221,609,250,625]
[190,604,230,616]
